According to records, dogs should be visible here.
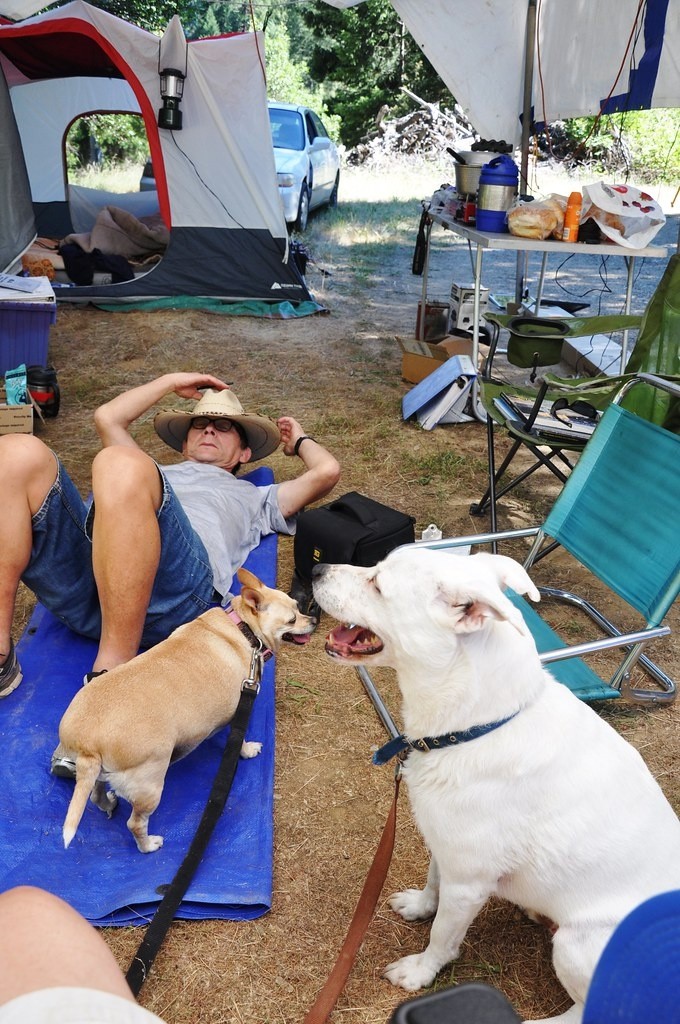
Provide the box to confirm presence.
[311,545,680,1024]
[59,567,318,854]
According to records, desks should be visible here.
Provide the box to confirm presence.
[420,203,668,425]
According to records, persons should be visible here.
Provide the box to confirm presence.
[0,886,166,1023]
[0,371,340,778]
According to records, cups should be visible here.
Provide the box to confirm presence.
[93,272,111,296]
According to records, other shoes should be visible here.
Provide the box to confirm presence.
[0,639,23,697]
[50,671,117,780]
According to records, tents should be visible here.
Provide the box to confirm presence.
[0,0,321,309]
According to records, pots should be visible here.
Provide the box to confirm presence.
[453,151,513,194]
[28,383,60,418]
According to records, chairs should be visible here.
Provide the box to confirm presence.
[279,123,300,145]
[469,251,680,564]
[349,373,680,762]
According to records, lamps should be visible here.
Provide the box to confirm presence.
[157,14,187,130]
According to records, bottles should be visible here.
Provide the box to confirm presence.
[476,155,518,233]
[562,192,582,243]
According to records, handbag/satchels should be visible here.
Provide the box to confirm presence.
[294,490,415,587]
[579,180,667,250]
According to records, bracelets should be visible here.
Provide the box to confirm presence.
[295,436,318,457]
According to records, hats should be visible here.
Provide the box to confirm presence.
[153,388,281,462]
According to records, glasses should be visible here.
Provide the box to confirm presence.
[551,398,598,428]
[190,416,233,432]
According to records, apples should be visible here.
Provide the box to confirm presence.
[23,258,55,281]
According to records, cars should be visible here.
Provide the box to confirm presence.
[139,102,340,233]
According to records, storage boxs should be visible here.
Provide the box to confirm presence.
[0,275,57,378]
[0,388,45,437]
[450,283,489,336]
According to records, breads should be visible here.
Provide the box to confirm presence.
[585,204,625,237]
[508,195,568,242]
